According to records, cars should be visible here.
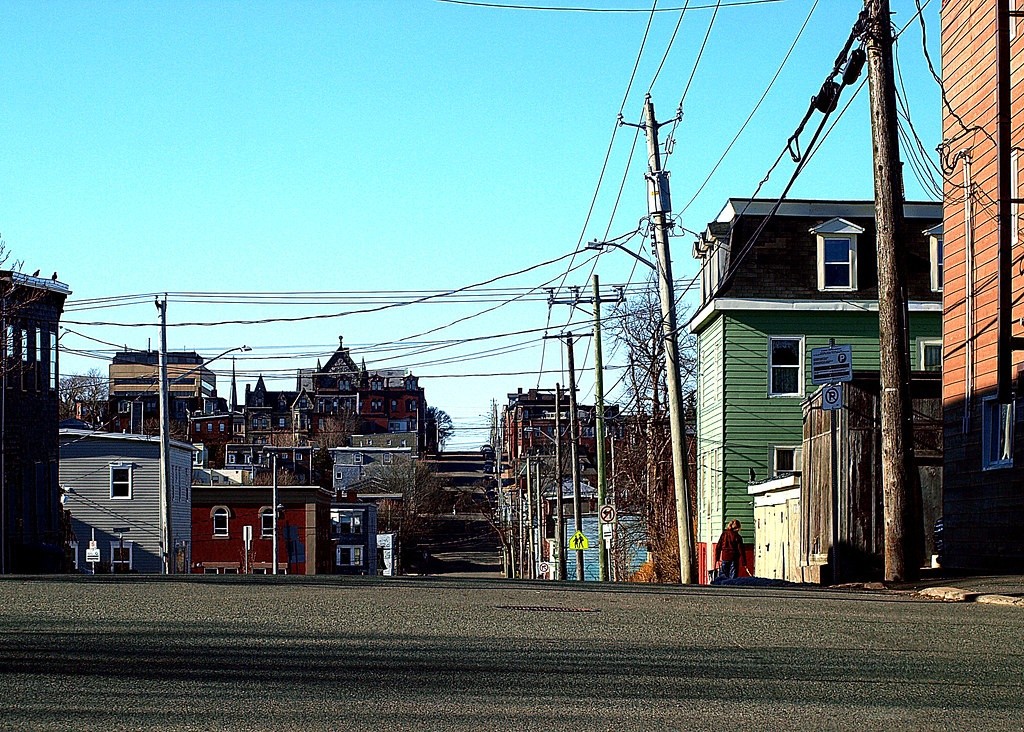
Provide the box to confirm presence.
[480,445,496,474]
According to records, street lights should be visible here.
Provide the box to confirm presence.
[523,426,565,581]
[585,237,693,585]
[158,345,253,574]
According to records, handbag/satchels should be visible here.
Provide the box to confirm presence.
[708,561,720,585]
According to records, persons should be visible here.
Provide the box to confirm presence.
[713,519,747,580]
